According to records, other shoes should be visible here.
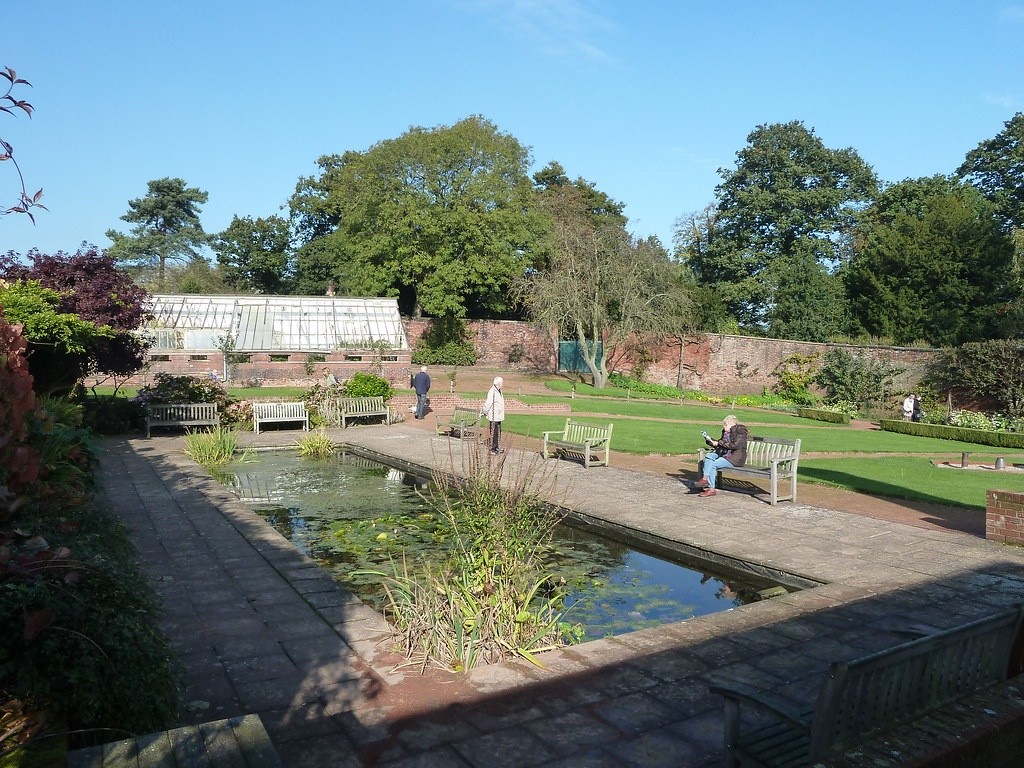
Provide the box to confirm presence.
[694,479,710,488]
[700,489,717,497]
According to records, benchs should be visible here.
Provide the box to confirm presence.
[253,401,310,434]
[336,395,390,429]
[435,405,483,440]
[543,418,614,469]
[696,436,802,508]
[145,402,222,440]
[707,600,1024,768]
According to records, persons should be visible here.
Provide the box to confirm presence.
[912,395,921,423]
[322,367,338,388]
[209,369,218,381]
[413,366,430,420]
[694,415,748,497]
[902,393,916,422]
[482,376,504,453]
